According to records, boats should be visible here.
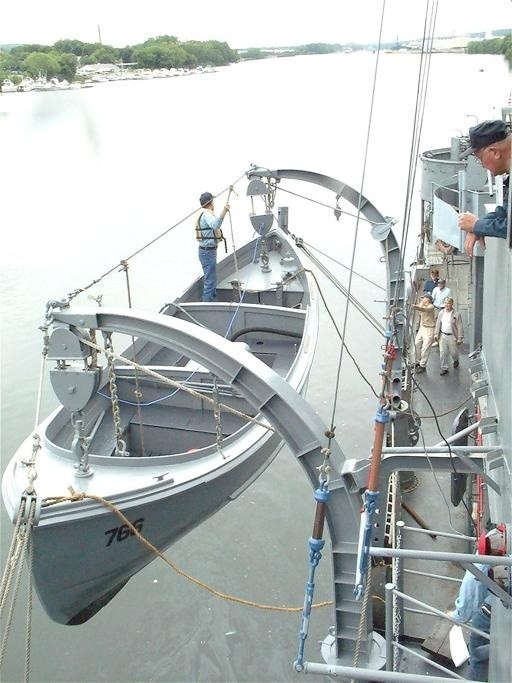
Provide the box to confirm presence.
[0,226,318,626]
[1,64,217,93]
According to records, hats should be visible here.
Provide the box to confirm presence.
[437,279,446,284]
[420,292,434,301]
[457,119,511,160]
[199,191,217,206]
[431,269,439,275]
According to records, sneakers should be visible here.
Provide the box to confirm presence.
[414,362,426,374]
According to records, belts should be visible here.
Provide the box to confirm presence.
[439,329,454,336]
[198,245,218,251]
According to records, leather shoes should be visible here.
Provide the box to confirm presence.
[440,368,449,377]
[453,360,460,368]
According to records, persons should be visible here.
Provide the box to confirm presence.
[410,269,464,375]
[446,549,491,680]
[457,119,512,257]
[195,193,231,302]
